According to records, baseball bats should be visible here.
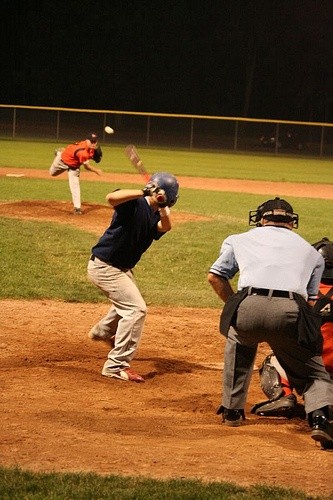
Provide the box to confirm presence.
[126,144,165,204]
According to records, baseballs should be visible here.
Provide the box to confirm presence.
[104,126,114,134]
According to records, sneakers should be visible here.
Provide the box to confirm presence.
[310,415,333,449]
[222,409,242,426]
[255,394,297,418]
[101,368,146,383]
[88,331,115,345]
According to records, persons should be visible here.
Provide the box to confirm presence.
[208,197,333,448]
[255,237,333,416]
[50,133,103,216]
[87,170,180,382]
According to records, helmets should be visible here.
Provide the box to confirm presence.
[148,172,179,208]
[311,237,333,278]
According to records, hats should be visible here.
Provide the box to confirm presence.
[261,199,293,214]
[88,132,98,142]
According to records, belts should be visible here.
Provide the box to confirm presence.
[251,287,294,299]
[90,255,95,261]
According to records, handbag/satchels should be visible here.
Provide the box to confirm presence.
[219,286,249,338]
[292,292,324,356]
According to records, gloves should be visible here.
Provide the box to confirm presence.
[142,181,159,197]
[151,189,168,208]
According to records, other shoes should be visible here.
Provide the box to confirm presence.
[54,148,64,156]
[74,208,83,215]
